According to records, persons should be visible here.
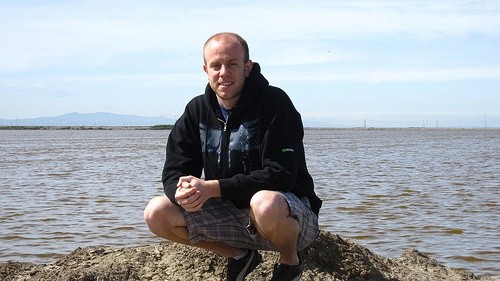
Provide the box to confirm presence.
[144,33,323,280]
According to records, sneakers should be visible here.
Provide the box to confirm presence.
[268,253,304,281]
[225,248,262,281]
